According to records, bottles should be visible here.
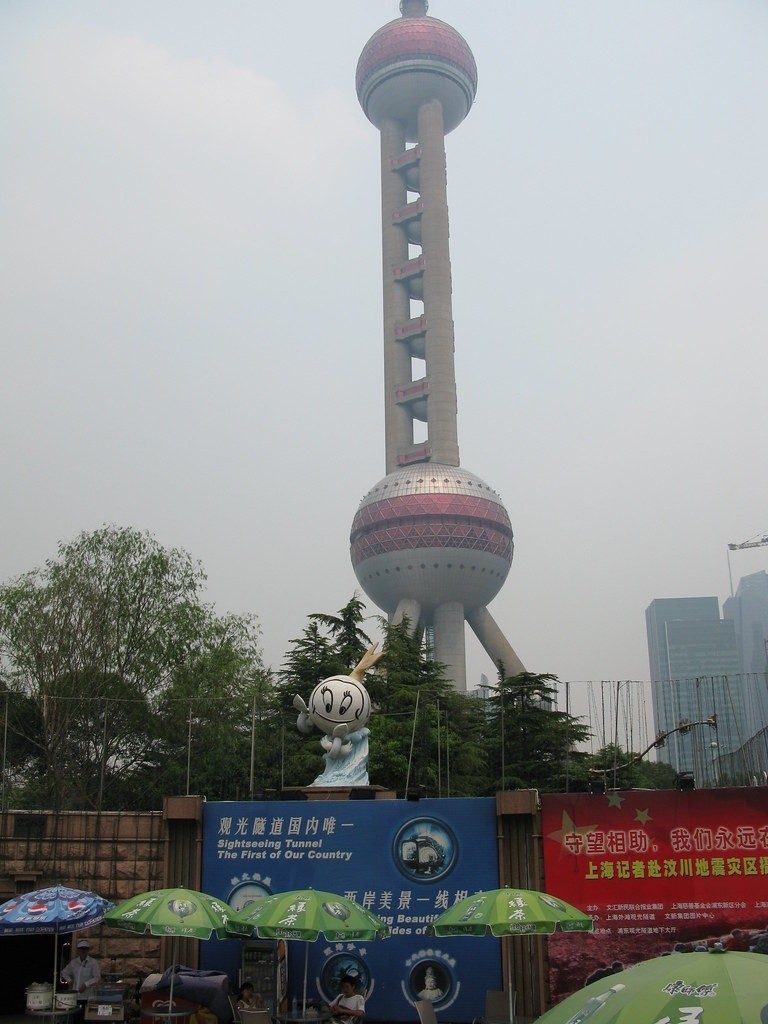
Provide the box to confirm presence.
[564,983,626,1024]
[245,951,276,964]
[241,897,278,921]
[458,896,485,921]
[246,976,273,991]
[352,902,384,928]
[292,995,297,1016]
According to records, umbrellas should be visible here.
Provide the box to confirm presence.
[425,889,594,1024]
[104,888,240,1013]
[225,890,391,1017]
[533,943,768,1024]
[0,885,117,1011]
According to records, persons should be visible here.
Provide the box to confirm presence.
[235,982,280,1024]
[61,940,100,1007]
[661,929,768,956]
[323,975,365,1024]
[586,961,623,987]
[417,968,443,1000]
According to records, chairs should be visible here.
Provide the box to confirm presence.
[473,990,517,1024]
[227,994,281,1024]
[414,999,438,1024]
[330,989,368,1024]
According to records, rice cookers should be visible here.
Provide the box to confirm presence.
[25,982,54,1012]
[55,990,81,1009]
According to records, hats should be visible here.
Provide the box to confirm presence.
[77,941,89,947]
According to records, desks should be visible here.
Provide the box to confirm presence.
[272,1011,330,1024]
[142,1008,198,1024]
[26,1008,81,1024]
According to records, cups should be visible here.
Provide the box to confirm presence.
[111,958,116,973]
